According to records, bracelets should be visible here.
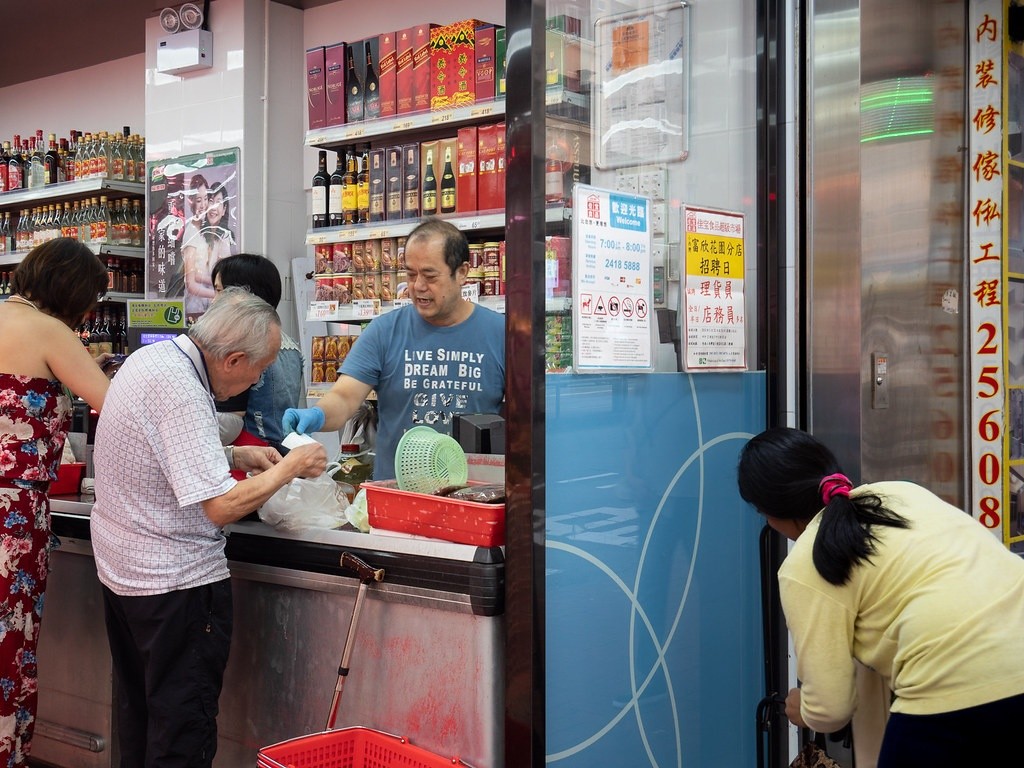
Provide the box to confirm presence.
[225,445,236,470]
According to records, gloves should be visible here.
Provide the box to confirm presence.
[282,406,325,438]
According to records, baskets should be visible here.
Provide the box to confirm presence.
[395,426,467,495]
[256,726,476,768]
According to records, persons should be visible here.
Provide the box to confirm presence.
[150,163,236,314]
[0,237,120,768]
[282,219,505,483]
[737,428,1024,768]
[89,287,327,768]
[211,254,305,455]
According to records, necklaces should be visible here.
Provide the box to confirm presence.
[4,295,39,310]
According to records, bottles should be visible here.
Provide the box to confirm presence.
[364,41,379,116]
[32,130,47,188]
[21,137,36,188]
[101,257,145,293]
[423,149,437,215]
[74,307,130,359]
[329,146,346,226]
[0,271,16,296]
[9,134,23,192]
[440,147,456,213]
[347,46,363,119]
[55,126,145,185]
[406,149,419,218]
[326,444,373,533]
[546,127,564,202]
[0,212,15,256]
[108,198,145,247]
[342,149,358,226]
[357,142,372,223]
[0,141,14,195]
[14,195,112,254]
[389,151,401,220]
[371,154,383,222]
[312,151,331,228]
[44,134,59,189]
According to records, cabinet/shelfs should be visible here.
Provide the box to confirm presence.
[0,176,145,303]
[305,90,572,399]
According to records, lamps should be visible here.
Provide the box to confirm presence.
[158,3,205,33]
